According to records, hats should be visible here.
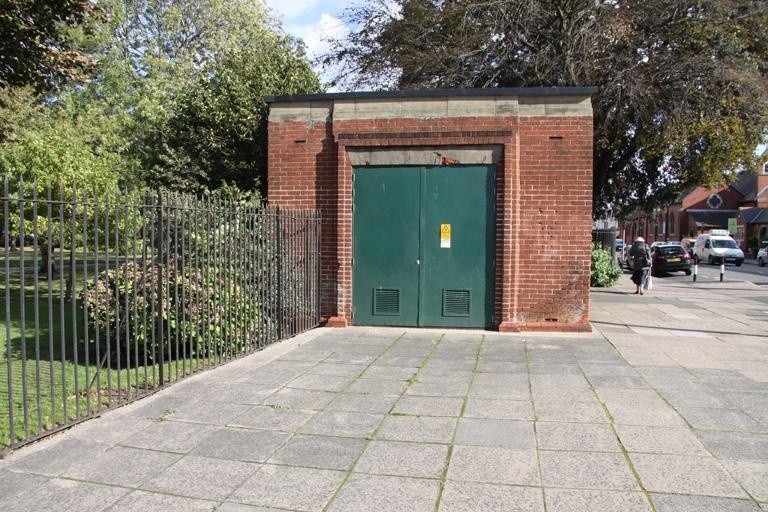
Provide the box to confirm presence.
[634,237,645,242]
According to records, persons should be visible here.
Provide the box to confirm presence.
[628,236,652,295]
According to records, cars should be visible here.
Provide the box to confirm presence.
[757,239,768,266]
[617,244,650,269]
[650,246,692,275]
[616,239,623,249]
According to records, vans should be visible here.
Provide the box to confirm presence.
[681,238,696,255]
[693,235,744,265]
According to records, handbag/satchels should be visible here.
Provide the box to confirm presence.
[643,276,652,290]
[643,255,651,267]
